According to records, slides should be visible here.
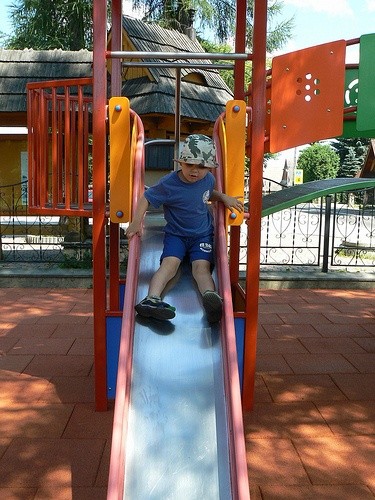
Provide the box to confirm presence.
[106,201,253,500]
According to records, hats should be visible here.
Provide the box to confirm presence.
[173,134,219,169]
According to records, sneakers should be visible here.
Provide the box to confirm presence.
[203,289,223,323]
[135,296,176,319]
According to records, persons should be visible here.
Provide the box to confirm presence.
[124,133,245,325]
